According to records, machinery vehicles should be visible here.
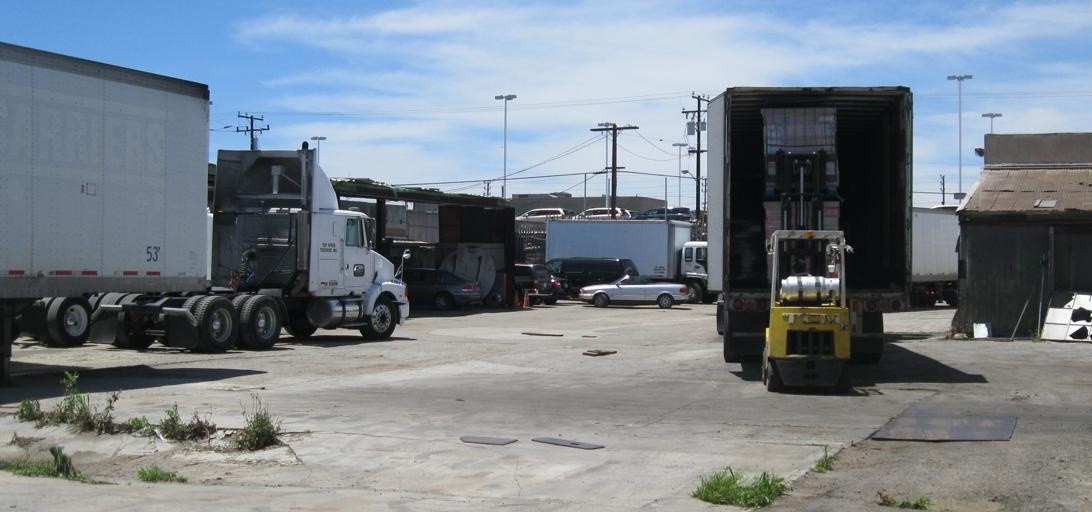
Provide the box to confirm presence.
[762,148,855,395]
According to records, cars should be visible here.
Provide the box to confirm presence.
[396,268,484,312]
[515,207,708,221]
[516,256,689,309]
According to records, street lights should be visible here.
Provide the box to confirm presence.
[947,73,974,210]
[681,169,701,221]
[494,94,517,198]
[981,113,1003,133]
[672,143,688,207]
[309,135,327,166]
[598,122,616,207]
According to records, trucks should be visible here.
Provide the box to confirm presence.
[546,220,717,304]
[0,41,410,387]
